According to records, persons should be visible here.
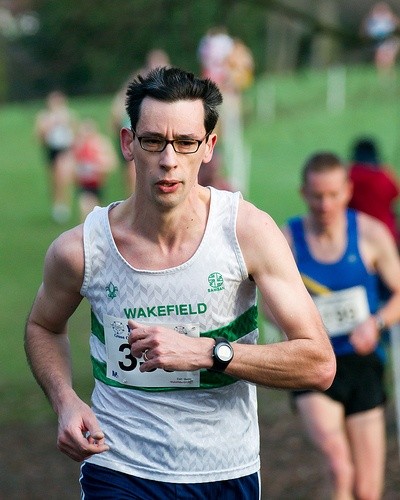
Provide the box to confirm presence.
[260,0,400,500]
[35,0,254,226]
[25,65,337,500]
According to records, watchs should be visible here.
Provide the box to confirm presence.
[207,336,234,375]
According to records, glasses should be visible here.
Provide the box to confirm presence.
[130,124,212,154]
